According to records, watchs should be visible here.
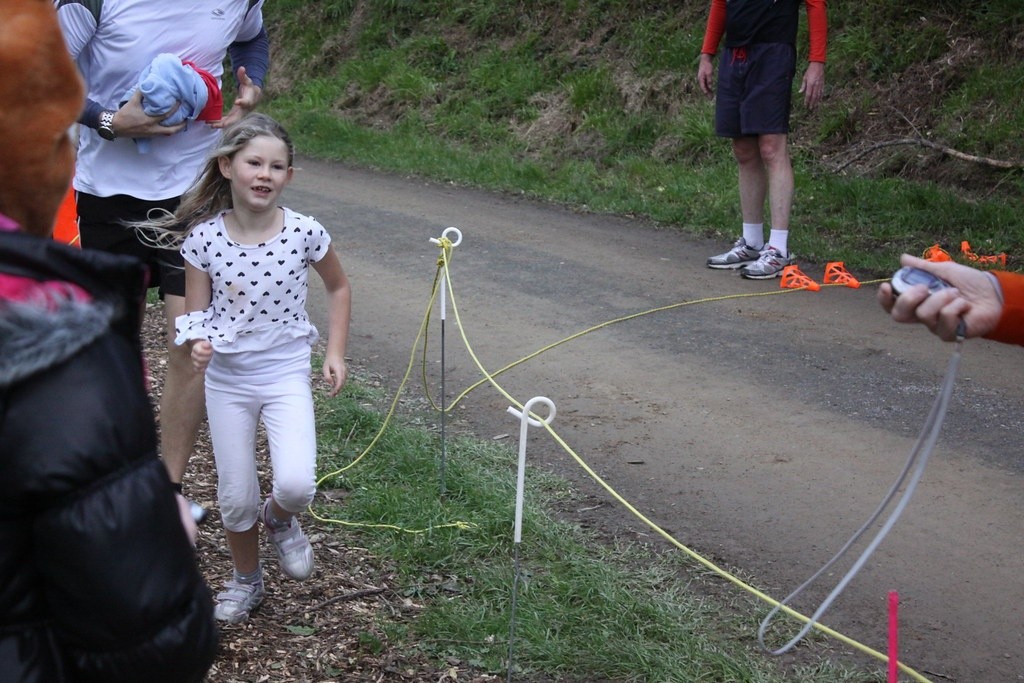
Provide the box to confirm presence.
[98,111,116,141]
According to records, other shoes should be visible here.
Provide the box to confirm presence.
[187,500,203,523]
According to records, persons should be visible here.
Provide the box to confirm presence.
[0,0,353,683]
[696,0,827,280]
[877,254,1024,344]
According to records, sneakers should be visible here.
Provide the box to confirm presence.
[741,243,793,279]
[215,581,265,622]
[261,499,314,580]
[707,237,766,270]
